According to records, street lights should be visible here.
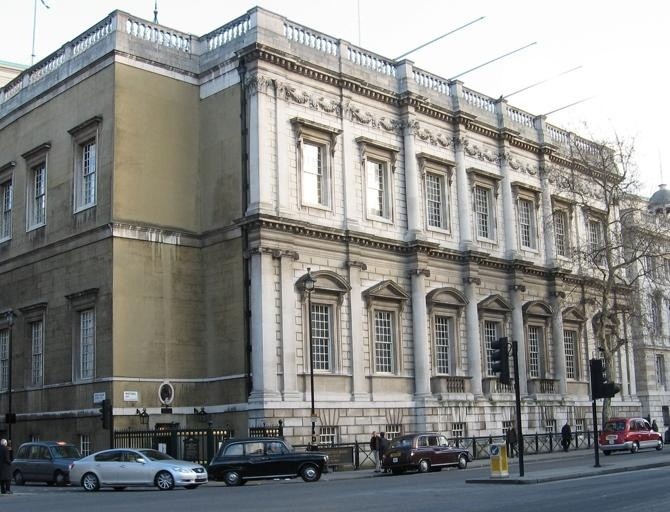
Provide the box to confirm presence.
[302,267,319,451]
[5,309,16,461]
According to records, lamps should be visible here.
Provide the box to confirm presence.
[136,407,149,425]
[194,408,208,422]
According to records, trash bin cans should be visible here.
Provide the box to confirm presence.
[664,424,670,444]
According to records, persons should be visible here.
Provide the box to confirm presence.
[0,437,15,494]
[507,424,519,458]
[652,419,659,432]
[369,430,382,472]
[561,420,573,452]
[379,431,390,473]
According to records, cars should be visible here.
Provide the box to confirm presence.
[598,416,663,456]
[12,441,82,487]
[207,438,329,487]
[68,447,208,490]
[379,433,473,474]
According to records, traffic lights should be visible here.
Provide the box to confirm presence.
[100,398,112,429]
[588,357,619,400]
[491,336,511,387]
